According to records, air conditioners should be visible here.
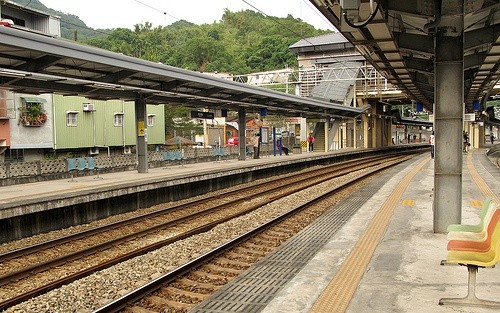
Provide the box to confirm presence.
[122,146,131,154]
[84,104,93,110]
[88,147,99,155]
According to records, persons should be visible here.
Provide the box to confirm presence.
[251,132,261,159]
[289,133,296,152]
[428,131,435,159]
[491,132,498,144]
[277,140,289,155]
[410,133,415,143]
[463,131,470,153]
[391,138,395,145]
[307,133,315,153]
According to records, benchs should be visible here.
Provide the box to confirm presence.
[68,158,102,182]
[213,149,229,163]
[161,151,186,169]
[439,196,500,310]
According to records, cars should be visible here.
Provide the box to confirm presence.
[226,137,240,146]
[188,141,214,149]
